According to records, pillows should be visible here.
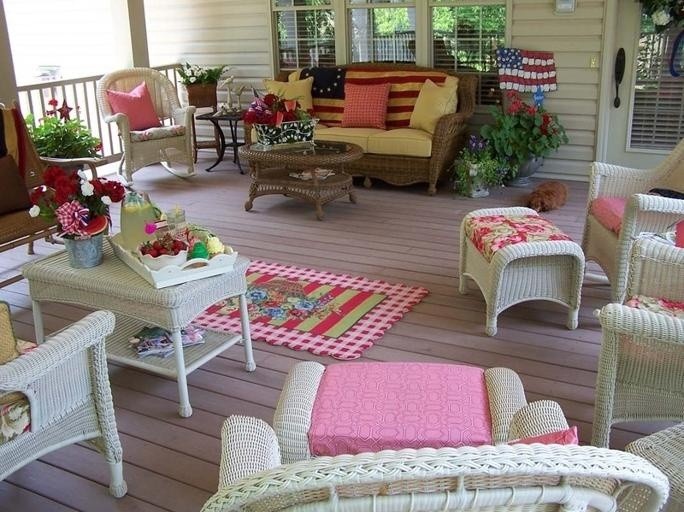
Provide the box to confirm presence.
[406,77,459,135]
[105,80,164,133]
[0,154,31,219]
[340,83,393,130]
[261,73,319,118]
[0,298,21,366]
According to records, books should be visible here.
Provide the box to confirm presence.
[124,326,206,358]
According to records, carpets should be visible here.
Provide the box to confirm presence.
[188,257,432,362]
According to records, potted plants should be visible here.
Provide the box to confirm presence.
[174,60,235,109]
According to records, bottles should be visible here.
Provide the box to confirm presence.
[120,191,157,252]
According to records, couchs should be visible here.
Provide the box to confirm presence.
[249,63,478,197]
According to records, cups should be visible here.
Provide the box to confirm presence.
[167,209,187,238]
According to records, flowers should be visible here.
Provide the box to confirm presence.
[638,1,684,37]
[241,84,317,128]
[219,285,341,329]
[479,88,570,161]
[24,165,128,239]
[449,132,518,198]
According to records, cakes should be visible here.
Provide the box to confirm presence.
[188,228,225,261]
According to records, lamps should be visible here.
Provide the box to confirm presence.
[552,1,577,16]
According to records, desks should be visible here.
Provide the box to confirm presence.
[21,236,258,420]
[191,110,248,175]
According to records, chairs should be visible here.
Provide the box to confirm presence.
[193,403,672,511]
[94,66,197,187]
[0,104,108,289]
[590,235,683,450]
[581,137,684,307]
[406,37,500,104]
[0,302,127,511]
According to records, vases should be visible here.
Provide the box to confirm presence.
[248,117,322,146]
[60,233,104,271]
[501,155,544,189]
[454,161,491,197]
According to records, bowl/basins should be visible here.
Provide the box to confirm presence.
[134,238,189,272]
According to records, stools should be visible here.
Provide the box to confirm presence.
[275,353,529,469]
[628,422,683,510]
[453,206,587,338]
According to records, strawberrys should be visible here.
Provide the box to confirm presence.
[141,234,187,255]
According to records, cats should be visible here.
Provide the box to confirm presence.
[523,180,568,214]
